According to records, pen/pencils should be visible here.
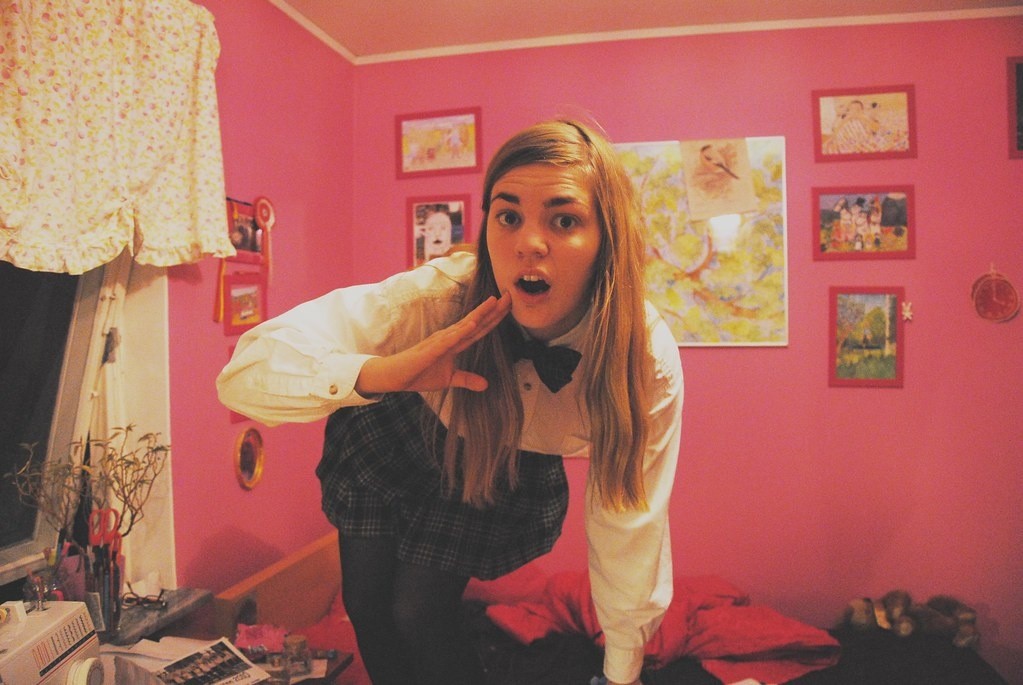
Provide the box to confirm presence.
[44,540,71,600]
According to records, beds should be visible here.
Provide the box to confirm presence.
[212,529,1007,685]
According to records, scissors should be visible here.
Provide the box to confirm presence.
[90,508,119,594]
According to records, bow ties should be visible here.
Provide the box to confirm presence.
[498,318,582,394]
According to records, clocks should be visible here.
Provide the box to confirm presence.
[973,273,1021,323]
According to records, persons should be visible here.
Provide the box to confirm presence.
[216,105,684,685]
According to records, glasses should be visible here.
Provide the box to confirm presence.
[122,581,169,610]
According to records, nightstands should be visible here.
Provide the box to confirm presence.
[239,648,354,685]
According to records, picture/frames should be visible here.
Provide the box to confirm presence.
[808,87,917,165]
[829,285,904,387]
[1007,56,1023,160]
[810,185,917,260]
[392,107,482,179]
[407,194,471,272]
